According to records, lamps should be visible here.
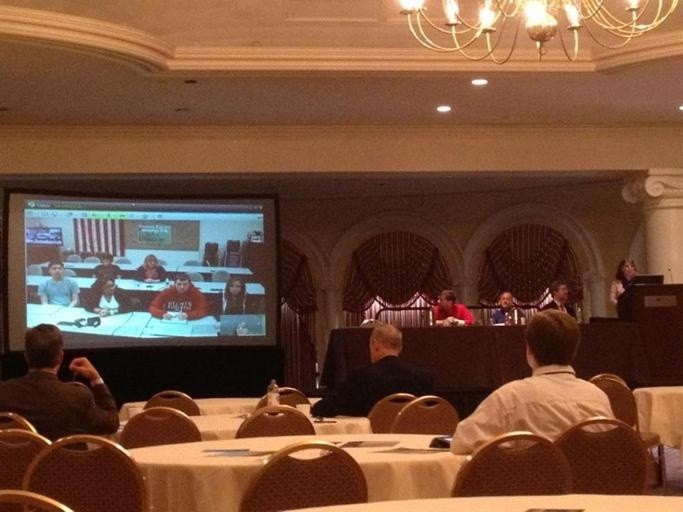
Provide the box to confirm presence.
[397,1,683,66]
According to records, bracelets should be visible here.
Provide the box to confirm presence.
[89,377,105,386]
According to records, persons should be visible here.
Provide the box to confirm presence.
[91,253,122,279]
[307,324,431,419]
[449,308,620,455]
[539,280,576,318]
[0,322,120,450]
[605,255,641,308]
[210,276,259,315]
[80,271,138,317]
[36,259,81,307]
[431,289,476,325]
[149,275,211,319]
[487,291,526,326]
[134,257,168,283]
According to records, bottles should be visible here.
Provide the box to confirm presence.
[512,307,521,325]
[576,304,582,326]
[267,379,280,408]
[427,307,434,327]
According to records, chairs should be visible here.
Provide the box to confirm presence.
[2,428,51,488]
[253,387,314,406]
[367,393,415,434]
[591,373,666,493]
[388,397,458,434]
[234,406,317,439]
[0,489,72,511]
[448,429,572,498]
[552,418,652,494]
[119,406,202,449]
[24,433,152,510]
[238,442,368,510]
[3,412,39,436]
[143,391,202,413]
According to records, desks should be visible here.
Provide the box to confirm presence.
[318,324,650,417]
